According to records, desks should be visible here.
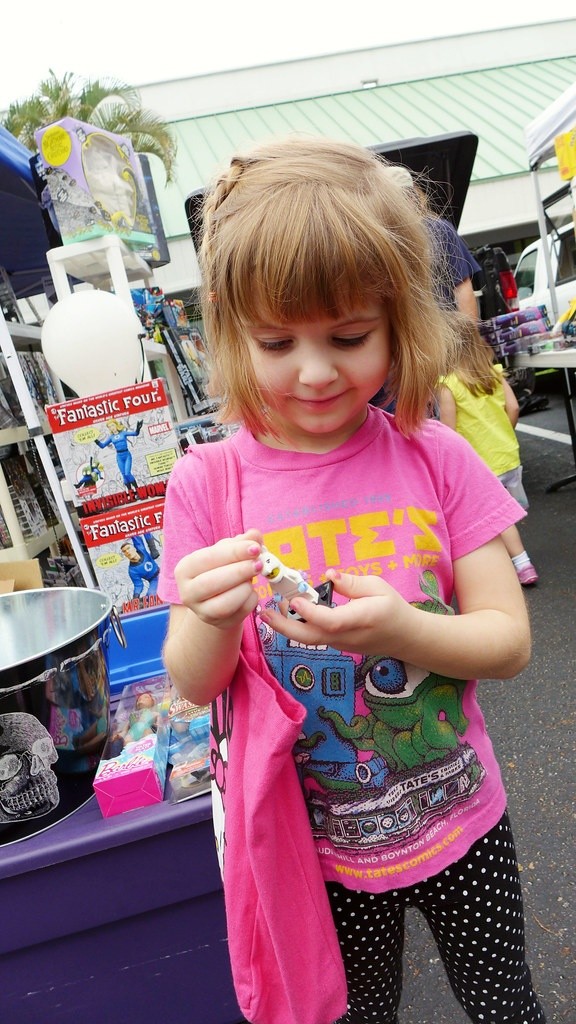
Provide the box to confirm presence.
[515,348,576,494]
[1,794,247,1024]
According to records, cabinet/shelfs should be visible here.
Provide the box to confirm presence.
[0,306,98,595]
[46,235,187,423]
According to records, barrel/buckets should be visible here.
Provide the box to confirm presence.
[0,586,127,847]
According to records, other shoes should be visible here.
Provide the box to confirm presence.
[518,563,538,585]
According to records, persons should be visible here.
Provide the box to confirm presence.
[368,164,538,589]
[156,139,546,1024]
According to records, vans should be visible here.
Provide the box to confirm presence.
[509,221,576,324]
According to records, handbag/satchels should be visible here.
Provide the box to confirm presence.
[208,441,348,1024]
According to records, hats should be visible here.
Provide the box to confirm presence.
[384,166,413,188]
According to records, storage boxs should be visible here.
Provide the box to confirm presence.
[1,116,225,614]
[479,304,555,368]
